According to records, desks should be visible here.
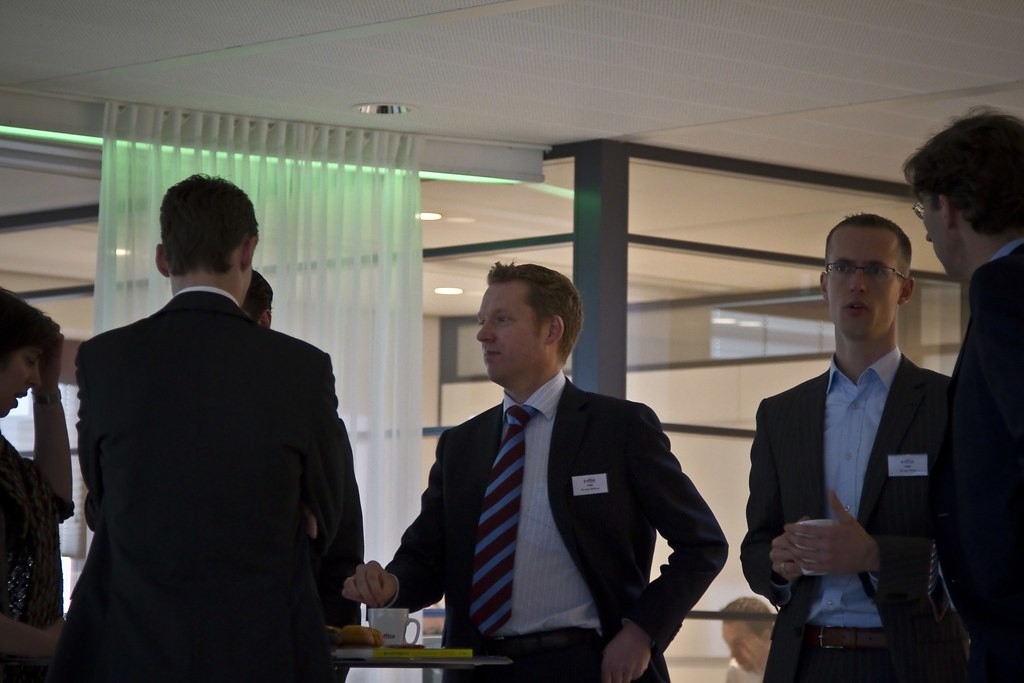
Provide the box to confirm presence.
[0,655,514,683]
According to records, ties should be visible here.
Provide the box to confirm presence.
[468,403,535,637]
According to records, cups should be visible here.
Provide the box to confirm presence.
[794,519,838,575]
[369,608,420,648]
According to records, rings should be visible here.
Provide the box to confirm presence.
[779,562,791,577]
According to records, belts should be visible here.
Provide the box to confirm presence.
[456,629,602,655]
[804,624,889,650]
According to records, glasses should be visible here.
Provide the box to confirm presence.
[827,262,907,280]
[913,196,939,219]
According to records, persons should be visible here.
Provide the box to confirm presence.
[740,214,953,683]
[719,597,778,683]
[0,286,73,683]
[45,173,368,683]
[901,113,1024,683]
[341,263,729,683]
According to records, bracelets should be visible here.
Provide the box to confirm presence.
[31,387,62,405]
